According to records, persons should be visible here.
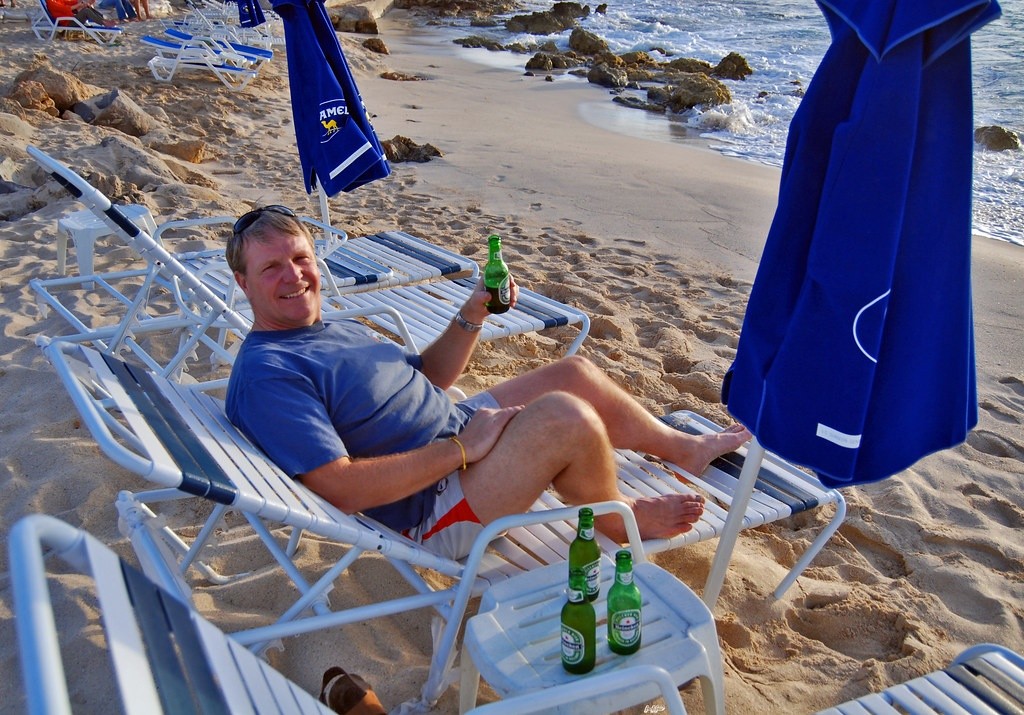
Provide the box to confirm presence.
[0,0,16,7]
[46,0,117,28]
[96,0,157,22]
[224,207,751,559]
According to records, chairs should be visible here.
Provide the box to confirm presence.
[5,514,1024,715]
[141,0,286,92]
[43,329,848,707]
[2,0,171,46]
[20,144,599,430]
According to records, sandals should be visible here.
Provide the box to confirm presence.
[320,667,388,715]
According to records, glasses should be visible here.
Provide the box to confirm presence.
[233,205,295,237]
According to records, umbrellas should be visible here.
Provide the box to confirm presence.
[267,0,390,233]
[237,0,266,44]
[702,0,1003,614]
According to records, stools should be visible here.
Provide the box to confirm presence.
[57,205,162,289]
[461,553,725,715]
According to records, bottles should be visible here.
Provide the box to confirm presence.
[607,550,641,656]
[483,235,510,314]
[560,508,601,675]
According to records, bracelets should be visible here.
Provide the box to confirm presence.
[456,311,483,331]
[452,437,466,470]
